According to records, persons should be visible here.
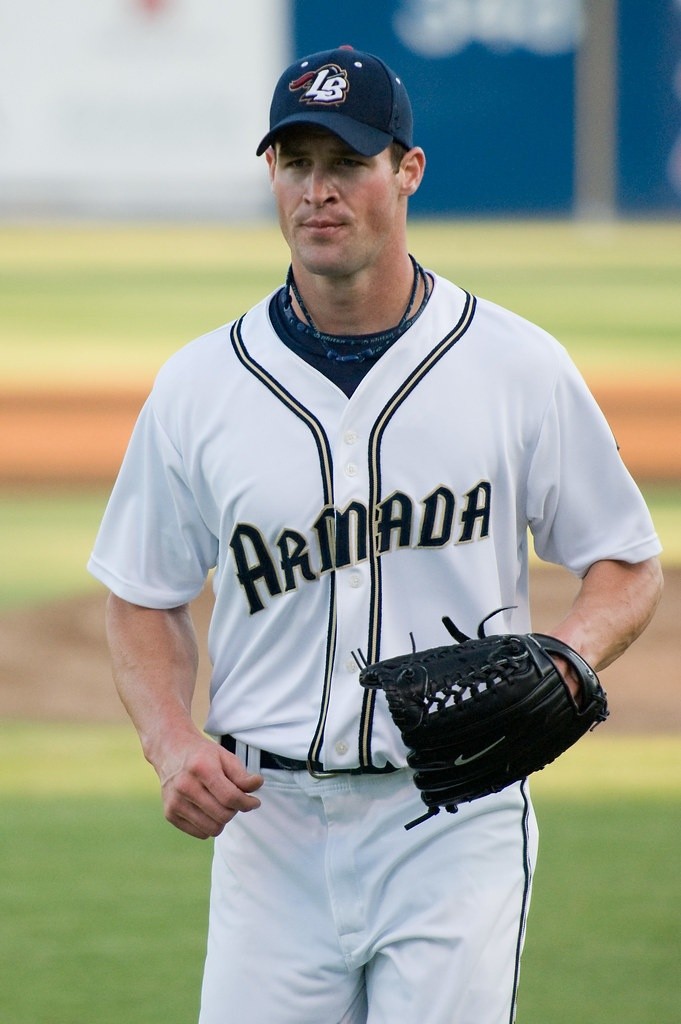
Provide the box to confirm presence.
[90,44,668,1024]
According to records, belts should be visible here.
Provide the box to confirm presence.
[216,733,395,776]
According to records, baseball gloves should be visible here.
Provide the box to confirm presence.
[358,633,612,816]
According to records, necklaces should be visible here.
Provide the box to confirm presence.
[283,253,430,362]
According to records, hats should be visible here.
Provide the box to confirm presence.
[254,43,417,160]
